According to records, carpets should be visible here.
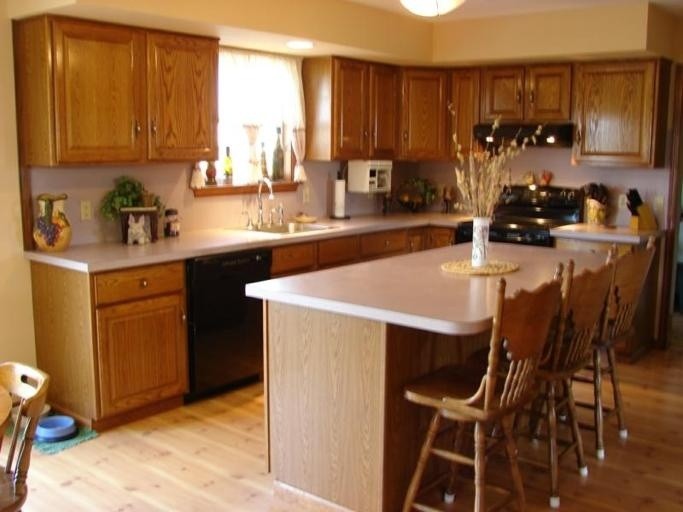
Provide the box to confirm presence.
[1,415,100,455]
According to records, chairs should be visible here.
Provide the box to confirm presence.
[468,244,618,511]
[399,260,563,511]
[533,235,655,460]
[0,362,50,512]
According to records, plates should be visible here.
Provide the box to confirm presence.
[292,215,318,223]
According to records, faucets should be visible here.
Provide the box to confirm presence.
[257,177,274,225]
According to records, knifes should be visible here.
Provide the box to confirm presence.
[625,186,645,216]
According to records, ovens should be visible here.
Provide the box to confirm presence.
[454,230,552,249]
[183,246,273,406]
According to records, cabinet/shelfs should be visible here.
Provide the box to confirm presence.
[299,56,401,165]
[272,227,410,276]
[483,64,570,125]
[9,13,220,169]
[25,251,190,431]
[570,58,672,169]
[450,69,480,161]
[401,66,449,162]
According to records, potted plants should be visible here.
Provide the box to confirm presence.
[95,173,167,247]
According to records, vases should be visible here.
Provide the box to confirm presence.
[471,216,491,268]
[30,191,73,252]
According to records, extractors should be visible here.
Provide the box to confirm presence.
[474,123,575,150]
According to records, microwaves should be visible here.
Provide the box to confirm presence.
[347,159,394,194]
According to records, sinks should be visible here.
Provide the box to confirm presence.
[223,221,340,235]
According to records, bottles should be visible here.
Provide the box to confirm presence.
[438,185,451,214]
[259,142,268,179]
[163,208,180,238]
[223,146,232,178]
[272,127,285,179]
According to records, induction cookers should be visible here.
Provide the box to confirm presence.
[455,214,573,239]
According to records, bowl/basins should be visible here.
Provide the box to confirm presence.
[32,416,77,442]
[9,403,51,430]
